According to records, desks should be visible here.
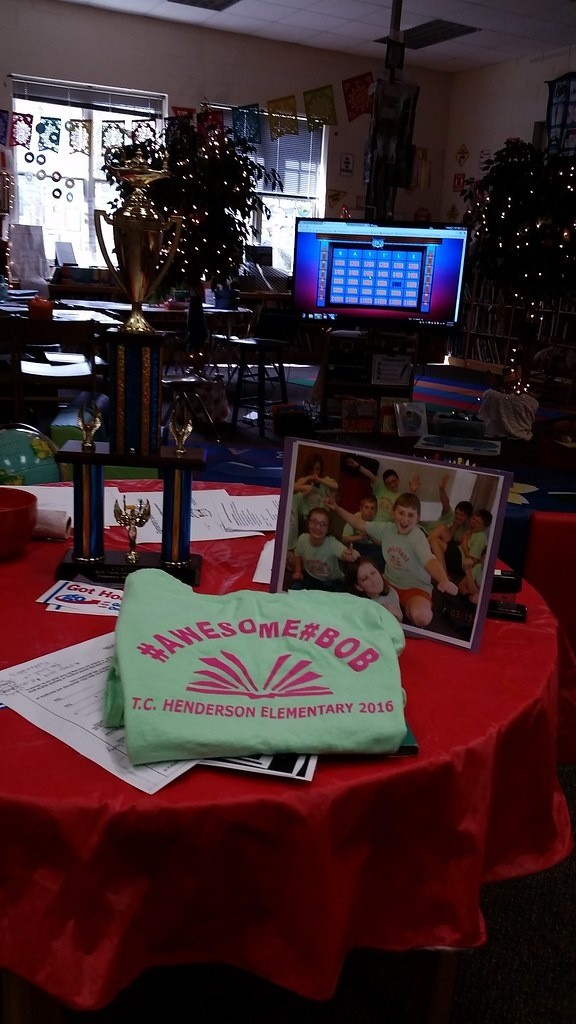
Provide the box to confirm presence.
[57,298,254,395]
[0,476,562,1024]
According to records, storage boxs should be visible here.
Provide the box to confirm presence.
[56,269,95,285]
[432,411,497,440]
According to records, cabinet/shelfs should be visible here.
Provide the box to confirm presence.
[49,284,310,362]
[441,263,576,384]
[0,312,96,424]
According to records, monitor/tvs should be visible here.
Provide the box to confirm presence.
[291,217,471,329]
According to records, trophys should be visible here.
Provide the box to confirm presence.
[165,405,194,453]
[95,147,186,333]
[77,400,103,452]
[115,497,151,563]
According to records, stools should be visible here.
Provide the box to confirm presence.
[229,337,290,441]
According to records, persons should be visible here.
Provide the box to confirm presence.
[283,453,492,643]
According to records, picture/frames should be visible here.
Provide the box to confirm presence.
[267,438,516,651]
